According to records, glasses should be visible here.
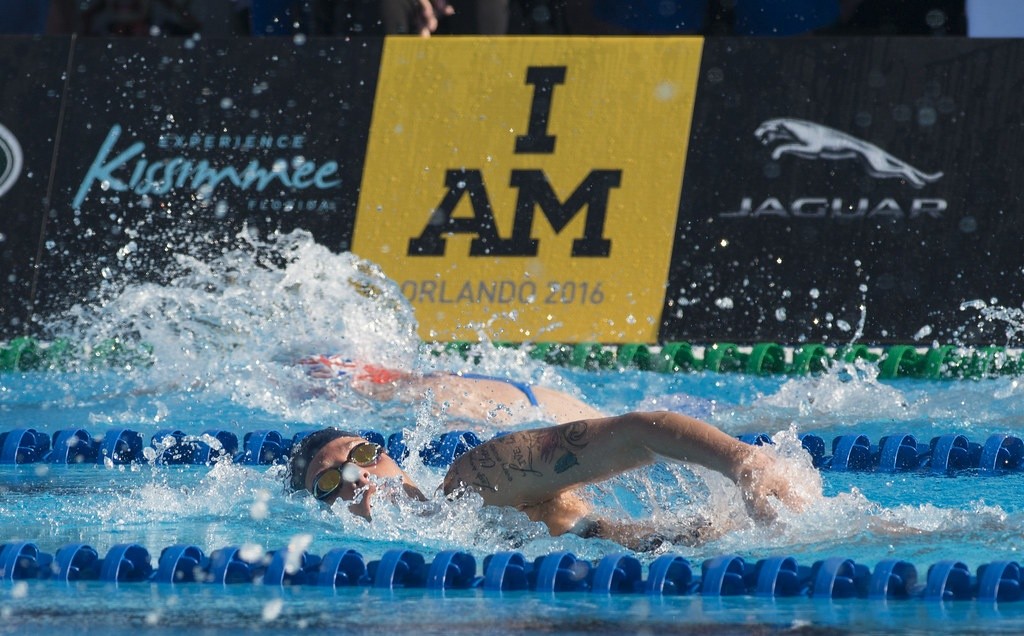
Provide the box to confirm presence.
[312,442,383,500]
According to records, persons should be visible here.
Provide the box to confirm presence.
[0,352,1024,557]
